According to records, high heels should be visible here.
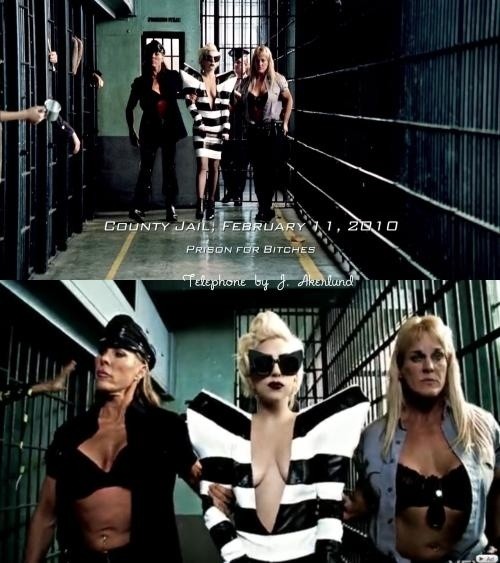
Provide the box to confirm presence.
[256,208,268,222]
[167,205,177,222]
[129,206,145,223]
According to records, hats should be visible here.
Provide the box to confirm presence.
[148,40,165,54]
[229,49,249,58]
[99,315,155,370]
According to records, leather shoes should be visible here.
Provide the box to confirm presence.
[222,192,233,202]
[234,197,243,205]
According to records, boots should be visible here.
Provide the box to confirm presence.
[196,198,203,222]
[206,197,215,220]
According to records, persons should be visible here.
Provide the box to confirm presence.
[0,360,76,407]
[185,310,369,563]
[342,314,500,563]
[0,36,104,154]
[125,38,294,225]
[24,314,235,563]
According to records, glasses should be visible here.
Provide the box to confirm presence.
[248,350,303,377]
[204,54,220,62]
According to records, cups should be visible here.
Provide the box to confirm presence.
[44,99,62,121]
